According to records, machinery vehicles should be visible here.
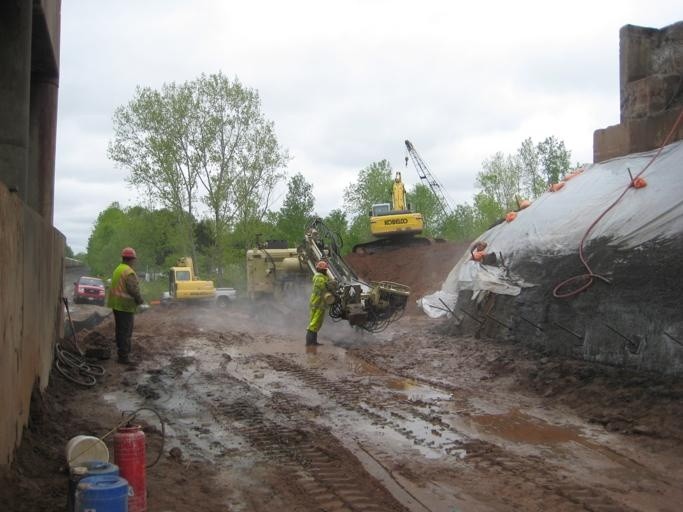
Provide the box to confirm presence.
[168,257,217,310]
[352,172,448,257]
[245,216,411,332]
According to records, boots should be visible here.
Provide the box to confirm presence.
[305,328,324,347]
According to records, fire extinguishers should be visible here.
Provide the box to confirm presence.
[58,408,164,511]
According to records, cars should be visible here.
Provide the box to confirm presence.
[72,276,106,306]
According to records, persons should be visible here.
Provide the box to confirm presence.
[305,261,332,346]
[107,247,143,364]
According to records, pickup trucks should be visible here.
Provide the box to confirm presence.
[158,280,238,308]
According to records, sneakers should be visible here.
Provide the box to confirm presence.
[117,354,137,365]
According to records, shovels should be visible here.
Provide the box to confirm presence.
[63,298,87,357]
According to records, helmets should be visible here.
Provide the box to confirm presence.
[120,247,139,259]
[316,260,329,270]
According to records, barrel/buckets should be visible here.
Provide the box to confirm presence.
[64,435,109,467]
[68,461,129,512]
